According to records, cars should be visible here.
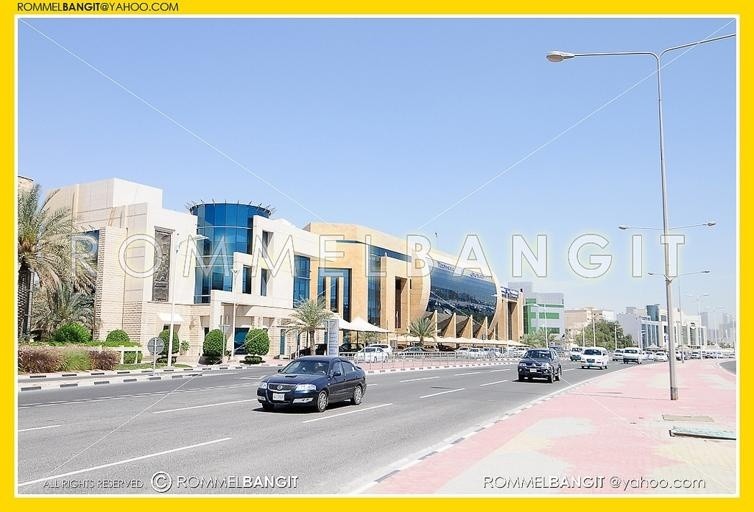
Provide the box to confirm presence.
[455,347,569,358]
[291,342,429,363]
[256,355,366,412]
[570,346,735,370]
[517,348,562,383]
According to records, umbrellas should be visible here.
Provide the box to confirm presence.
[397,334,525,347]
[271,316,395,350]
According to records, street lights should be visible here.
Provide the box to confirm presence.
[617,220,733,363]
[230,268,240,366]
[164,235,208,371]
[546,33,737,400]
